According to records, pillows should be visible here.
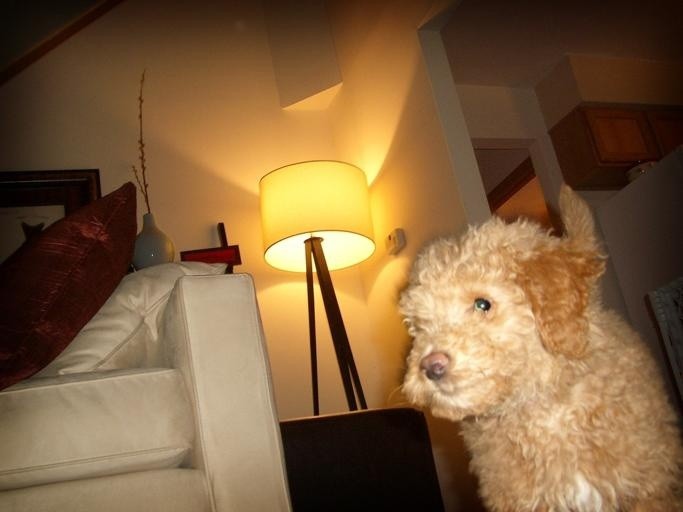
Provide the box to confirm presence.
[56,261,228,375]
[0,182,137,391]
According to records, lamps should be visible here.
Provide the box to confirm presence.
[259,158,377,416]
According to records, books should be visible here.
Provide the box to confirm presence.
[645,278,681,406]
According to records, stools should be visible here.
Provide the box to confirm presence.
[277,408,445,512]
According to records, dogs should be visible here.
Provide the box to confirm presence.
[389,182,683,512]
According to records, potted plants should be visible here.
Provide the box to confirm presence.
[130,64,175,269]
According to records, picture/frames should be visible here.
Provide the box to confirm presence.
[0,169,102,265]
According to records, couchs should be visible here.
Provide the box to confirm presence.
[0,273,293,512]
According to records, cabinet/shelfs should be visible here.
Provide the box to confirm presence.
[549,103,683,190]
